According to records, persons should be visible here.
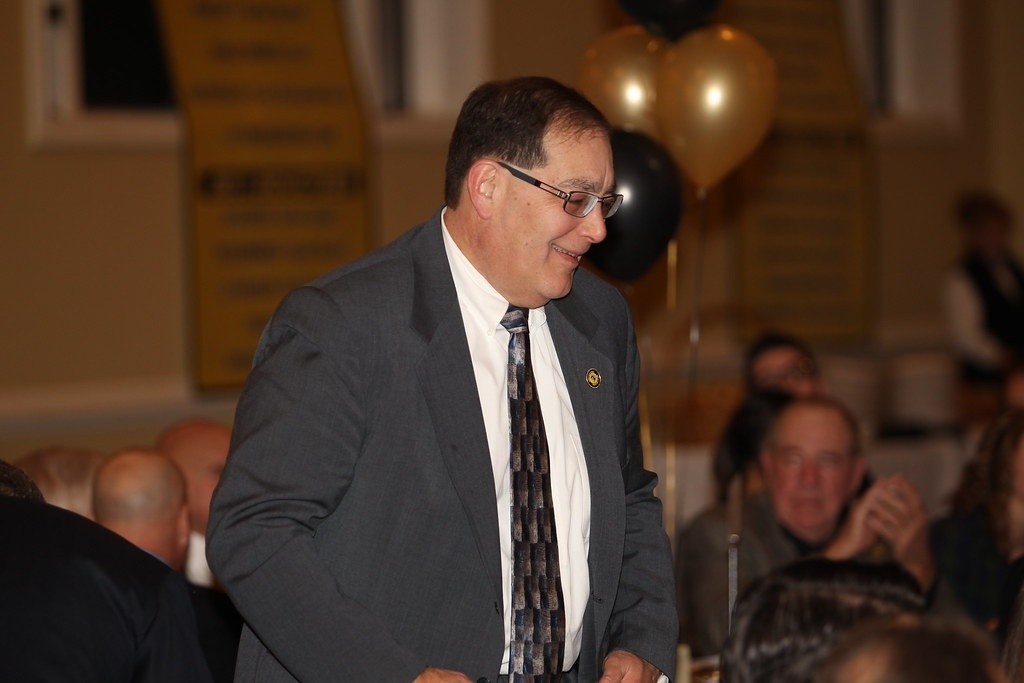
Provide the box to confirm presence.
[206,79,677,683]
[0,422,243,682]
[675,184,1024,683]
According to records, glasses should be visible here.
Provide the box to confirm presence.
[497,161,624,220]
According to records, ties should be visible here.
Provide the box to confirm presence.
[500,304,570,683]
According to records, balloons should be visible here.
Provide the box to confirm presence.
[580,1,779,280]
